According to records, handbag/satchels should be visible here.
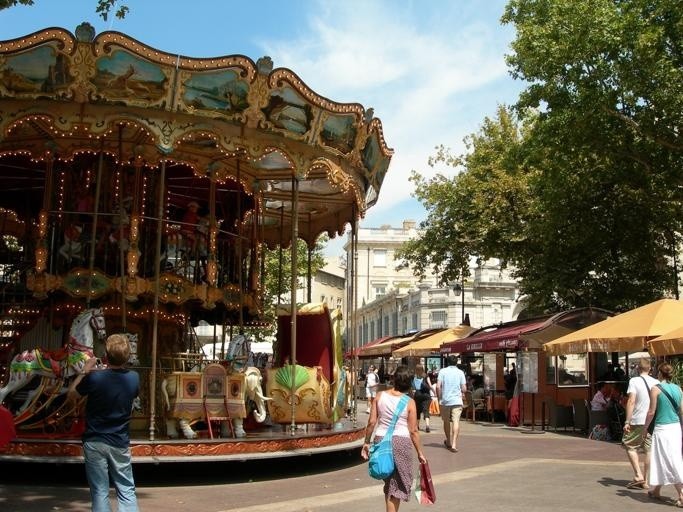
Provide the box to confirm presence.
[588,425,608,441]
[428,399,440,415]
[414,460,436,506]
[646,412,655,436]
[420,382,430,393]
[367,435,394,481]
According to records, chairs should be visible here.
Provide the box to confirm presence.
[355,381,395,401]
[547,398,573,432]
[465,390,485,423]
[585,401,619,436]
[572,399,589,433]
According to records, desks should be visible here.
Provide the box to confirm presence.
[484,396,508,423]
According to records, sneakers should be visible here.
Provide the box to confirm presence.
[425,428,429,432]
[365,408,370,414]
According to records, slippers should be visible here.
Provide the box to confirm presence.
[443,439,453,448]
[626,479,644,488]
[450,446,458,452]
[640,482,649,489]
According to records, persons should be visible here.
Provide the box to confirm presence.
[642,362,683,506]
[437,355,485,452]
[361,366,426,512]
[77,195,112,250]
[621,358,659,488]
[363,365,438,431]
[67,334,140,511]
[590,383,613,442]
[182,201,200,257]
